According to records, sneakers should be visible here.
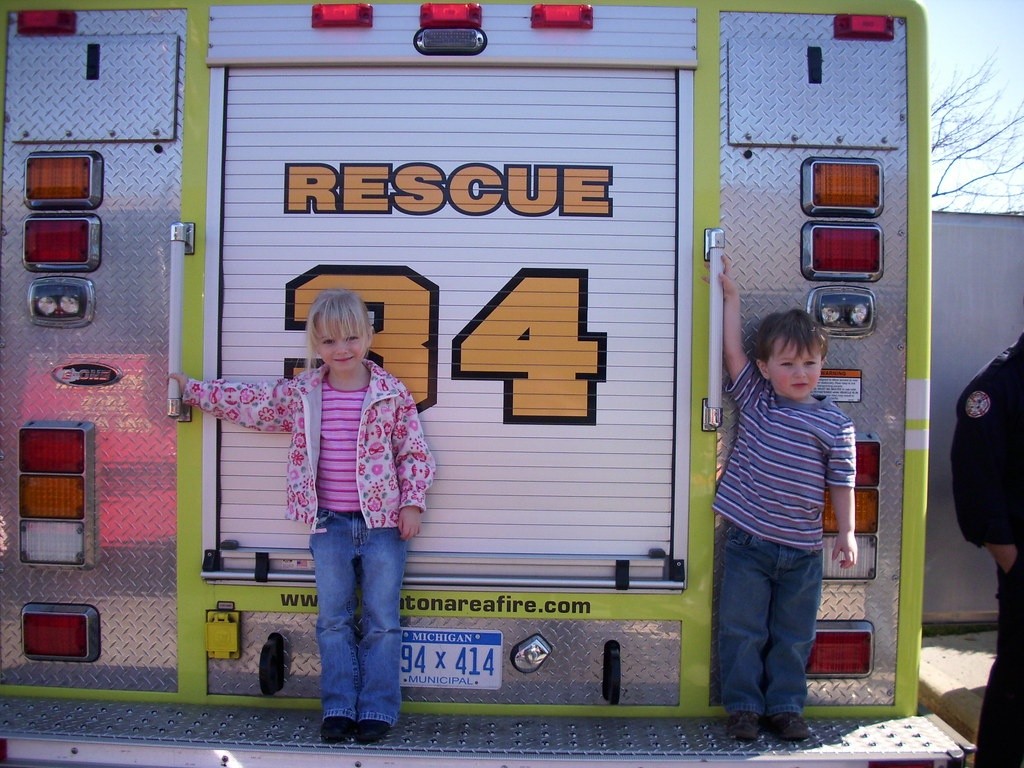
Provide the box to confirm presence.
[767,713,809,741]
[727,711,760,740]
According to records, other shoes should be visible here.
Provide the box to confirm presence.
[321,717,355,743]
[355,719,391,744]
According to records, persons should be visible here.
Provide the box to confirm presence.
[702,256,858,741]
[951,332,1024,768]
[165,289,436,744]
